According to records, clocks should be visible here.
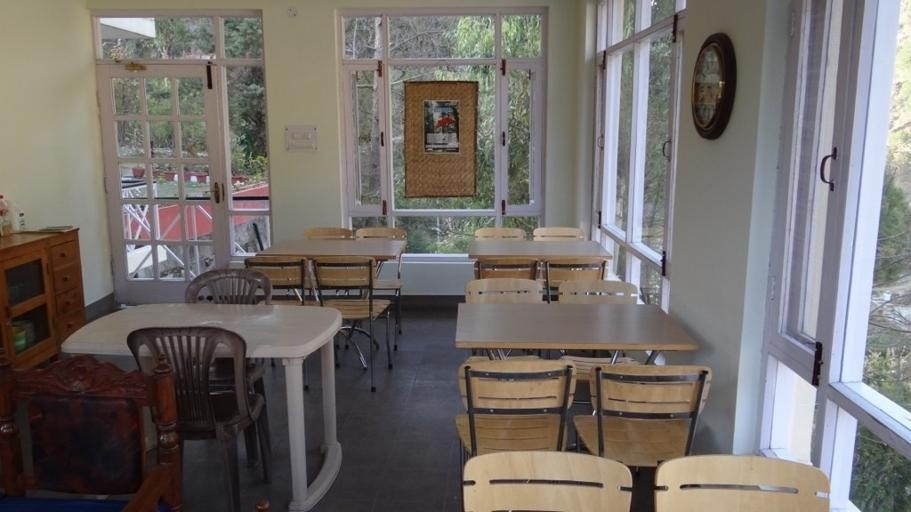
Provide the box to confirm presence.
[690,33,736,140]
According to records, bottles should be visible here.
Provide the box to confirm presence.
[17,213,27,233]
[0,195,14,237]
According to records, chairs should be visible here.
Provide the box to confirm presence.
[1,355,183,512]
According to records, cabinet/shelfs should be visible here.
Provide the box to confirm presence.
[0,227,87,373]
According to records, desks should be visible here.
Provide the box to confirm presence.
[61,303,343,511]
[120,177,163,251]
